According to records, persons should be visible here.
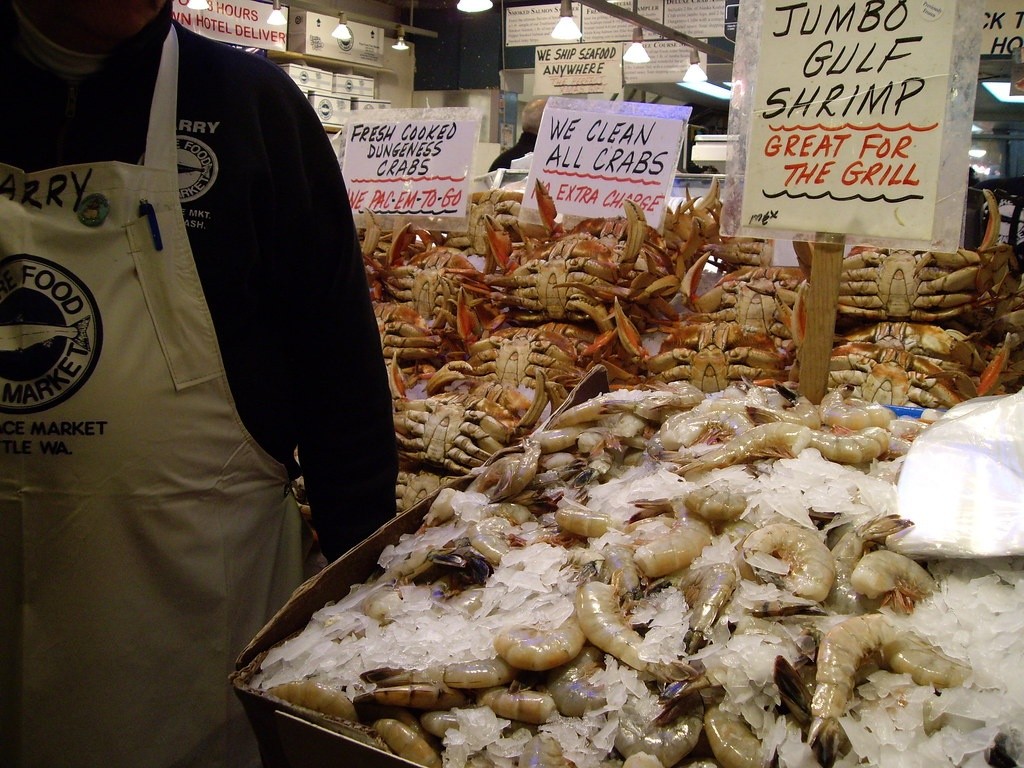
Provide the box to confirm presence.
[488,98,547,172]
[1,0,396,768]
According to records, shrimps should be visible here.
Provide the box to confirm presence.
[260,379,1011,768]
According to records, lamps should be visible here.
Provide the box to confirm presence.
[266,0,287,25]
[391,24,410,50]
[550,0,583,40]
[968,140,987,158]
[622,27,650,63]
[683,50,708,82]
[331,11,352,39]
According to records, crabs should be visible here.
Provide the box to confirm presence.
[287,171,1024,521]
